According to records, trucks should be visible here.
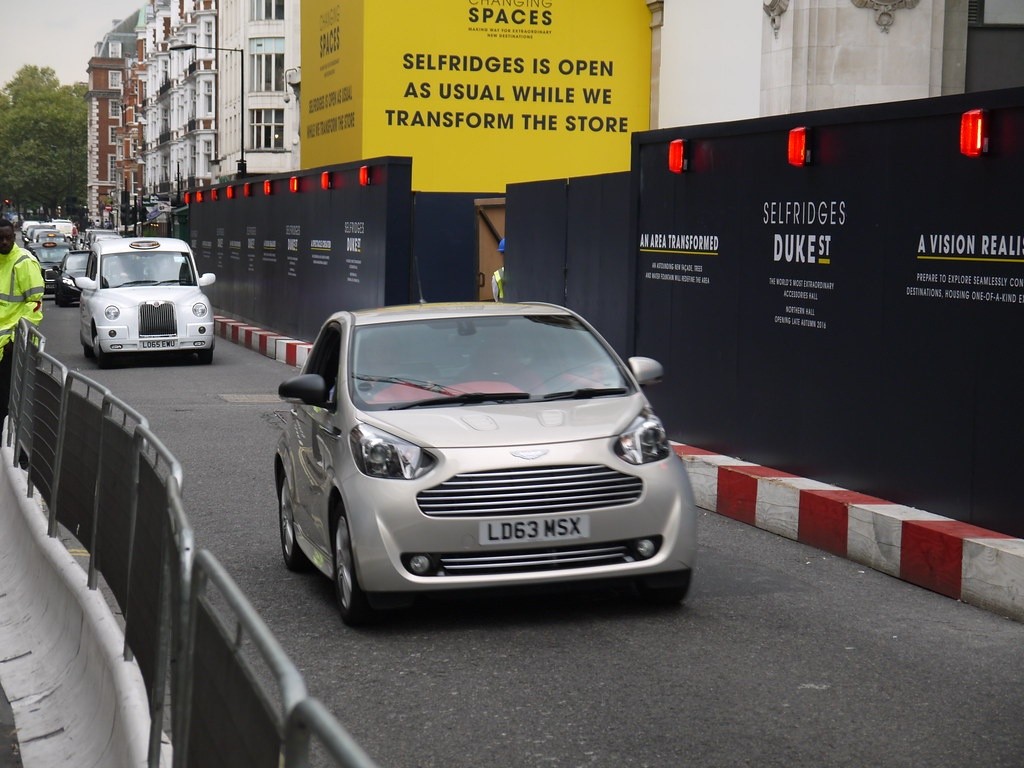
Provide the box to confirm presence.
[94,218,111,228]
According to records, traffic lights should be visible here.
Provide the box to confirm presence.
[120,190,129,226]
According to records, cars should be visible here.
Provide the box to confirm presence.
[18,217,80,243]
[36,232,72,243]
[269,298,699,629]
[51,249,92,307]
[84,228,124,249]
[27,242,75,294]
[74,239,218,368]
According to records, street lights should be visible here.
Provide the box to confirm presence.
[168,41,254,177]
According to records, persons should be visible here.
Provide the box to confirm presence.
[0,219,46,443]
[490,238,505,302]
